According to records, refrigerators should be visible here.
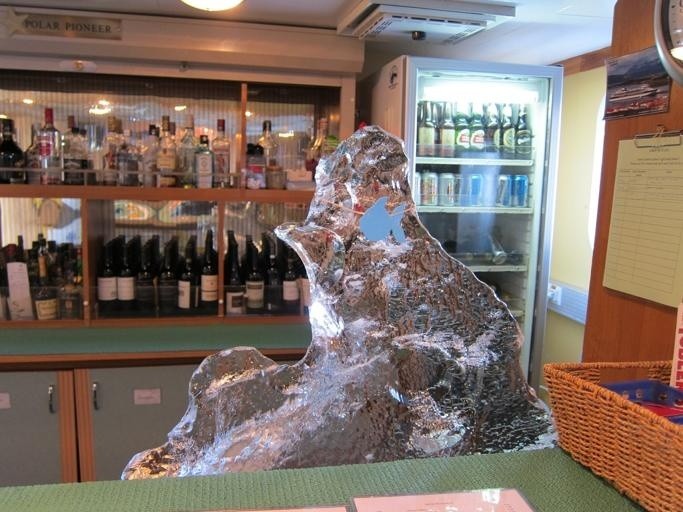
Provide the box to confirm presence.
[365,53,565,389]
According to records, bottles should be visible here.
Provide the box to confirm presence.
[223,229,310,316]
[0,231,82,320]
[243,119,285,190]
[97,230,219,315]
[415,95,531,159]
[304,117,339,187]
[0,105,232,189]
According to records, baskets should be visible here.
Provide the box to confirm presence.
[544,360,683,512]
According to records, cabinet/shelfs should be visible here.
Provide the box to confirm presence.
[0,6,365,333]
[0,360,199,485]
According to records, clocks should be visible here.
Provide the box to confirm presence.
[653,0,683,84]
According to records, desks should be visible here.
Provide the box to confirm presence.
[0,444,660,512]
